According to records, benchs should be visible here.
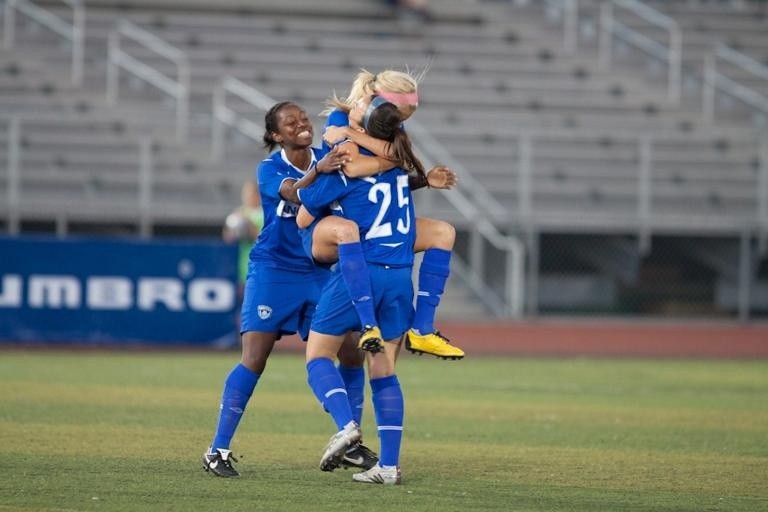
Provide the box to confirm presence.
[1,1,768,321]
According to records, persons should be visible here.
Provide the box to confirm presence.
[298,96,416,484]
[297,68,465,361]
[202,102,380,477]
[221,182,267,287]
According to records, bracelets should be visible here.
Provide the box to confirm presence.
[313,162,320,172]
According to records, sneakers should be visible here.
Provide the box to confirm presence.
[341,445,379,470]
[203,448,240,478]
[405,330,464,360]
[320,420,362,471]
[352,460,401,486]
[359,326,385,354]
